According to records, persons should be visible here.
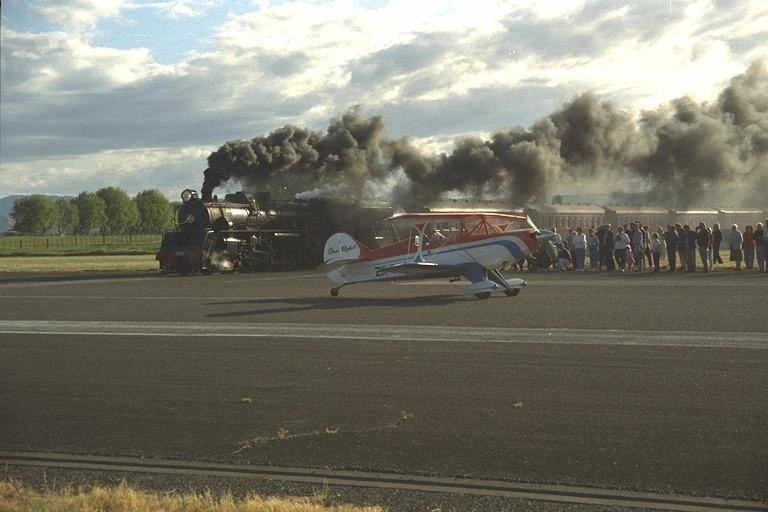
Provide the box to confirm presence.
[510,217,768,273]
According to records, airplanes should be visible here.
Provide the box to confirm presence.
[321,210,557,300]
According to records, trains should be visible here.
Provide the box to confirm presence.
[154,187,768,280]
